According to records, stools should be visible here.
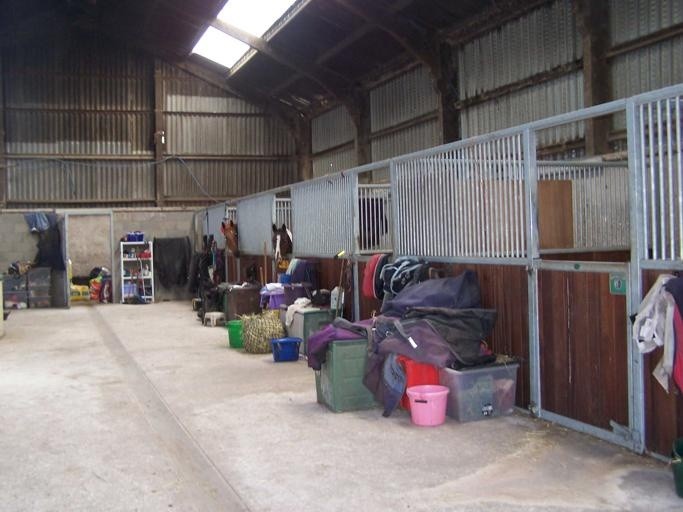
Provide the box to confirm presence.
[204,311,227,328]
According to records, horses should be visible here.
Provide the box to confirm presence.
[272,223,292,265]
[220,220,241,258]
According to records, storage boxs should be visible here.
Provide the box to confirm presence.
[314,336,522,424]
[1,267,54,311]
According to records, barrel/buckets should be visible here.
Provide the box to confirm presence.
[405,385,450,428]
[192,298,201,311]
[272,336,302,361]
[223,319,244,348]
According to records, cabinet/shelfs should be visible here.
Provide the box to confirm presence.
[119,240,155,304]
